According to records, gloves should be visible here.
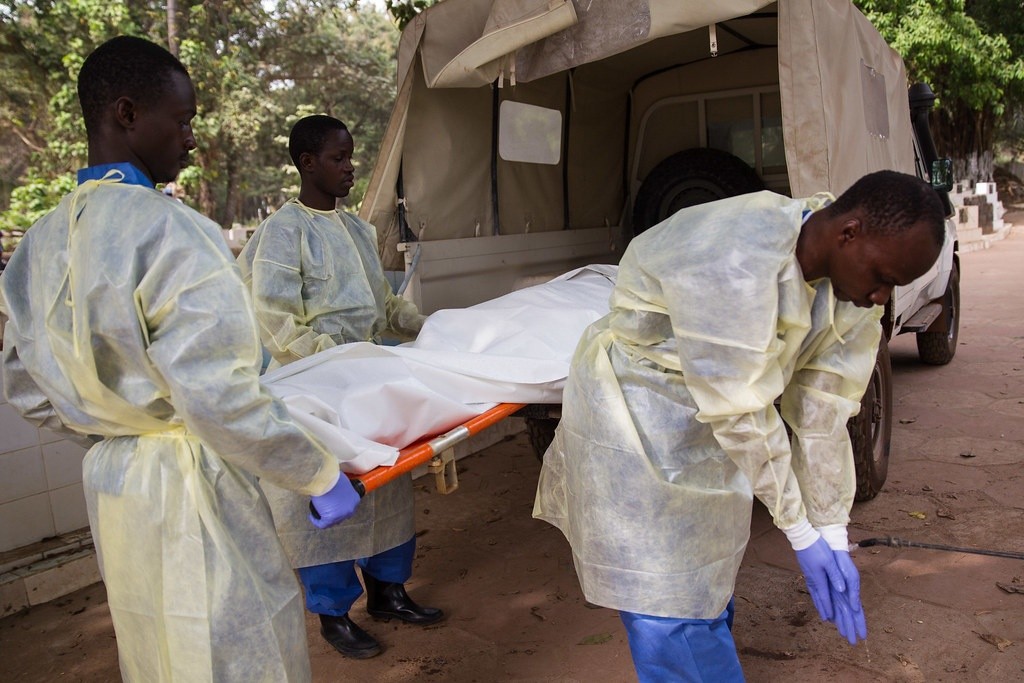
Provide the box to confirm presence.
[306,469,360,527]
[794,537,867,643]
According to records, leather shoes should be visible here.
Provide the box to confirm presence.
[361,569,443,625]
[319,613,381,659]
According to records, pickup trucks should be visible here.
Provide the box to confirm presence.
[397,0,958,499]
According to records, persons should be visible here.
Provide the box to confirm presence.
[233,113,454,662]
[533,169,947,681]
[2,34,361,683]
